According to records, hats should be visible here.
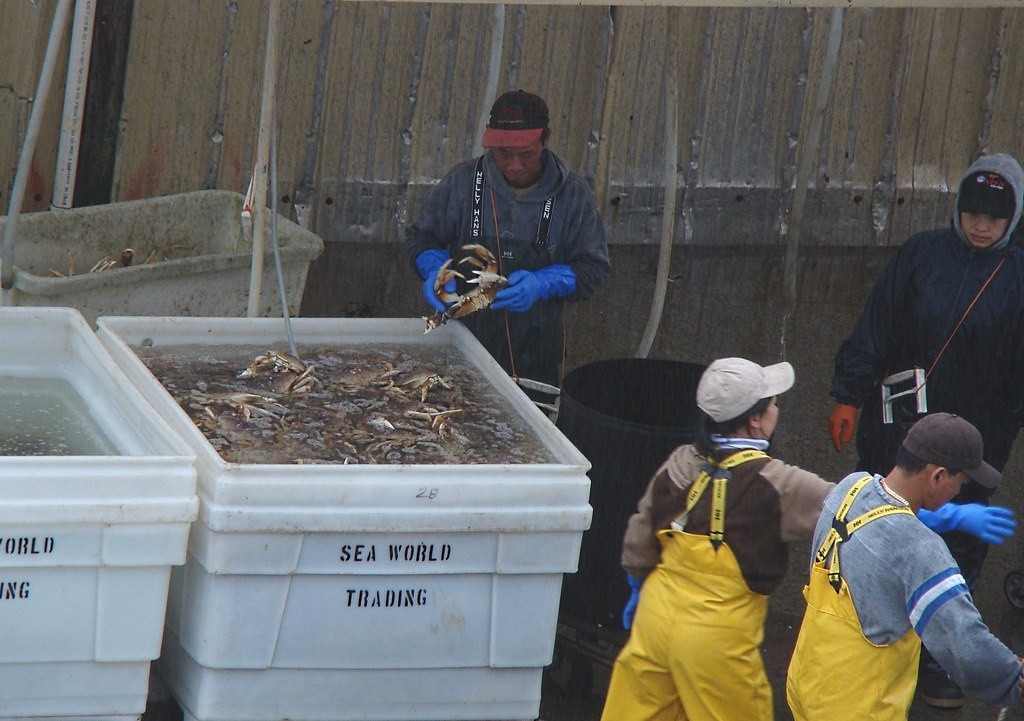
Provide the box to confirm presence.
[903,413,1002,489]
[958,170,1015,218]
[696,356,794,423]
[481,89,549,148]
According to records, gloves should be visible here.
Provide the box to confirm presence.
[414,248,456,312]
[828,403,858,451]
[622,573,644,631]
[490,264,576,313]
[915,500,1018,545]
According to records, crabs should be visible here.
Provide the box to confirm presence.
[127,341,567,466]
[422,242,507,337]
[45,244,188,284]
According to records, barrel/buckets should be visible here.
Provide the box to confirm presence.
[555,358,774,624]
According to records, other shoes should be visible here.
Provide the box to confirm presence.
[958,562,980,595]
[919,653,963,707]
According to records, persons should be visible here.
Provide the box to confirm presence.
[602,357,1017,721]
[408,88,613,416]
[785,412,1024,721]
[828,154,1024,709]
